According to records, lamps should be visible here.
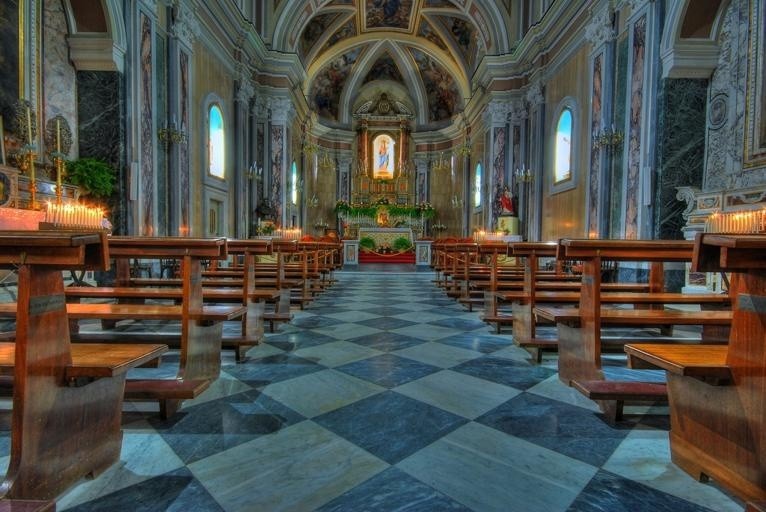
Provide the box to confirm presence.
[515,163,535,184]
[248,160,263,181]
[592,121,622,149]
[157,114,187,145]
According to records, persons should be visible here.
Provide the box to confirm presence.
[499,186,514,212]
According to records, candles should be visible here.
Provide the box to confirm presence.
[271,227,302,241]
[45,201,103,230]
[473,230,505,243]
[56,120,61,153]
[26,104,33,152]
[703,208,766,235]
